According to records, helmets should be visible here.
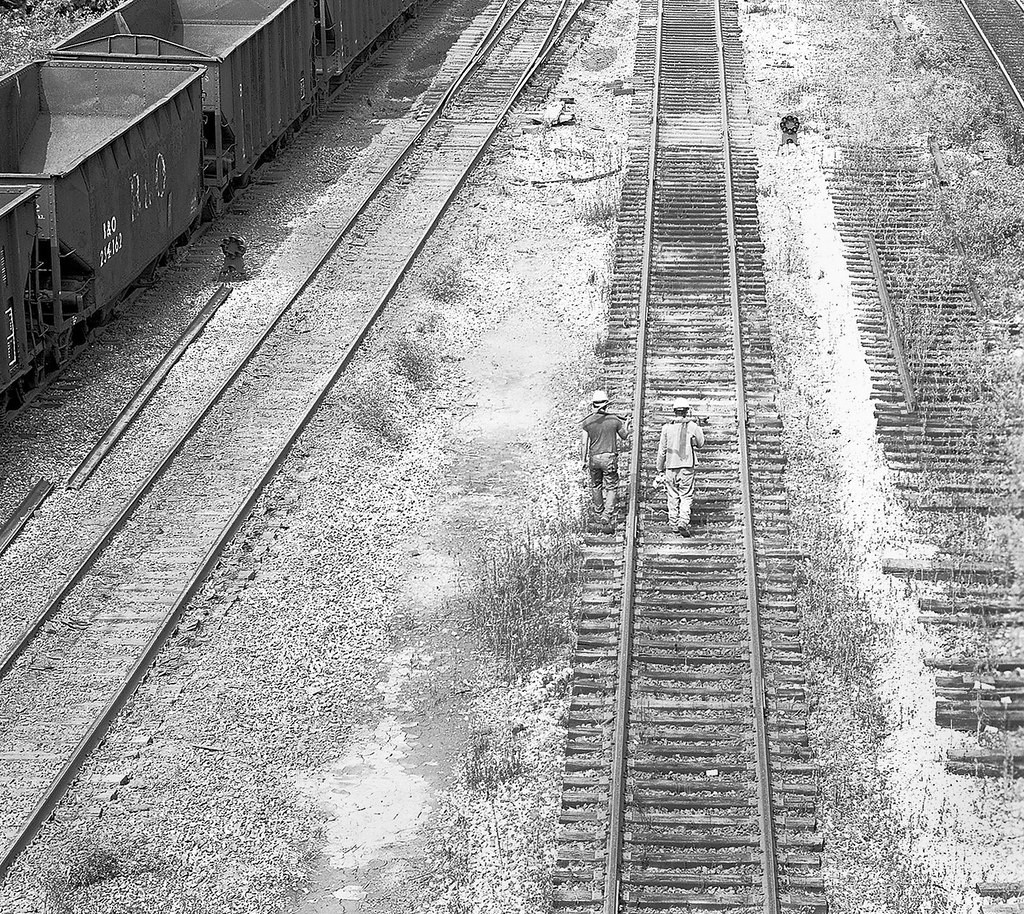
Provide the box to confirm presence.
[673,398,690,410]
[593,391,608,402]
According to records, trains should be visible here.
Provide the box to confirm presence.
[1,2,434,409]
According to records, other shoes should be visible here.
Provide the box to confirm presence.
[678,523,690,537]
[673,528,679,533]
[593,516,602,523]
[601,517,613,533]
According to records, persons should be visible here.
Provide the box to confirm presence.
[580,389,632,534]
[656,397,704,537]
